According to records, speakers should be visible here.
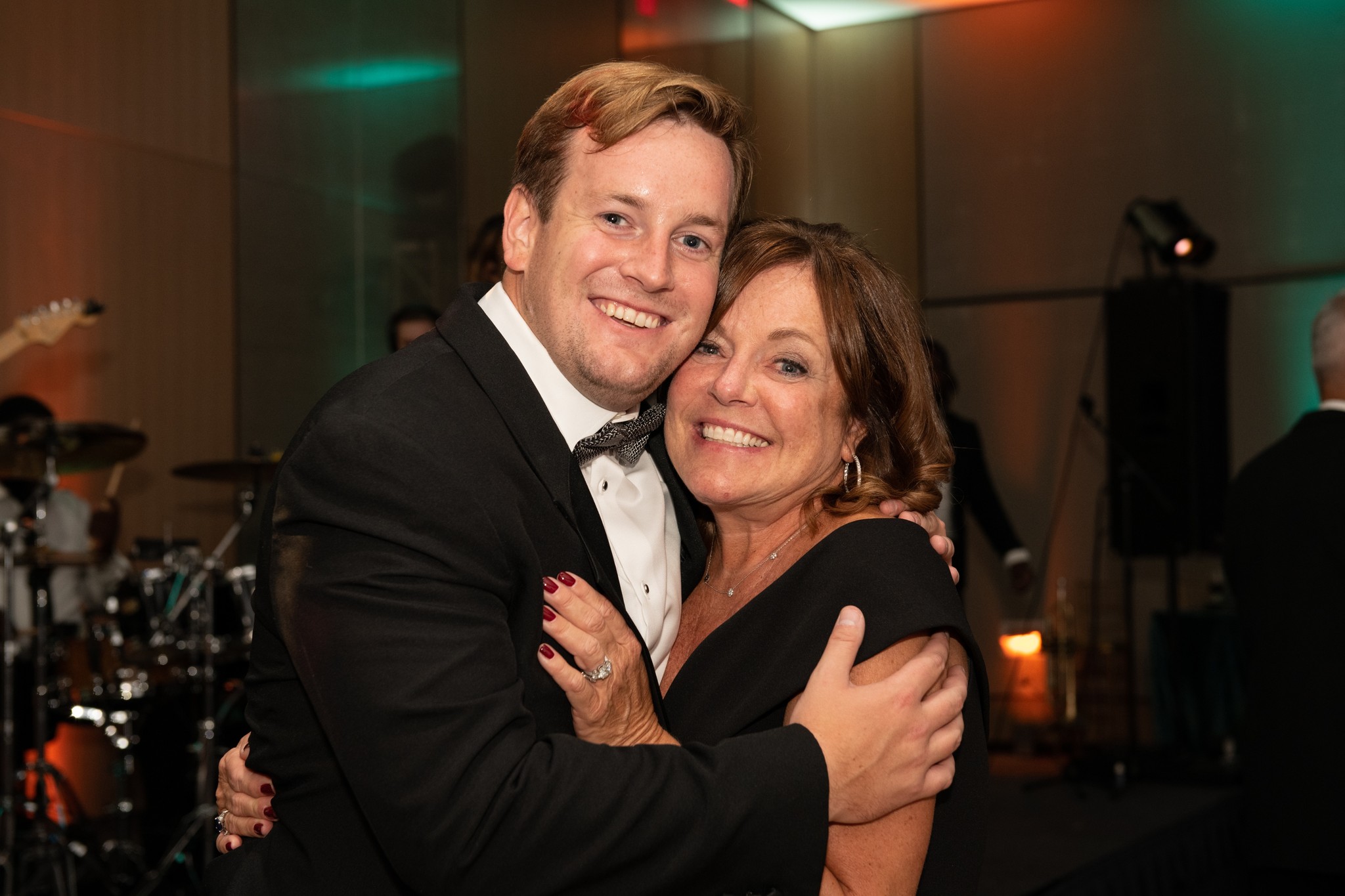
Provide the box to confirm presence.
[1107,279,1232,559]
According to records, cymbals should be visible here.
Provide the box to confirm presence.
[170,458,273,479]
[2,418,149,472]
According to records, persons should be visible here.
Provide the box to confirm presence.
[198,57,1038,896]
[1,392,141,667]
[385,303,434,361]
[1220,290,1344,896]
[162,537,243,657]
[209,213,973,896]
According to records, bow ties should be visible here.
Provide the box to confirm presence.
[572,403,666,466]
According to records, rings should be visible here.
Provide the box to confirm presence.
[581,659,612,683]
[215,810,230,834]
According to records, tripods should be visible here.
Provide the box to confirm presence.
[0,453,263,895]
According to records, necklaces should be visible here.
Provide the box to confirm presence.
[704,513,822,598]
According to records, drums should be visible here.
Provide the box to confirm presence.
[51,613,170,702]
[140,569,260,646]
[25,705,149,823]
